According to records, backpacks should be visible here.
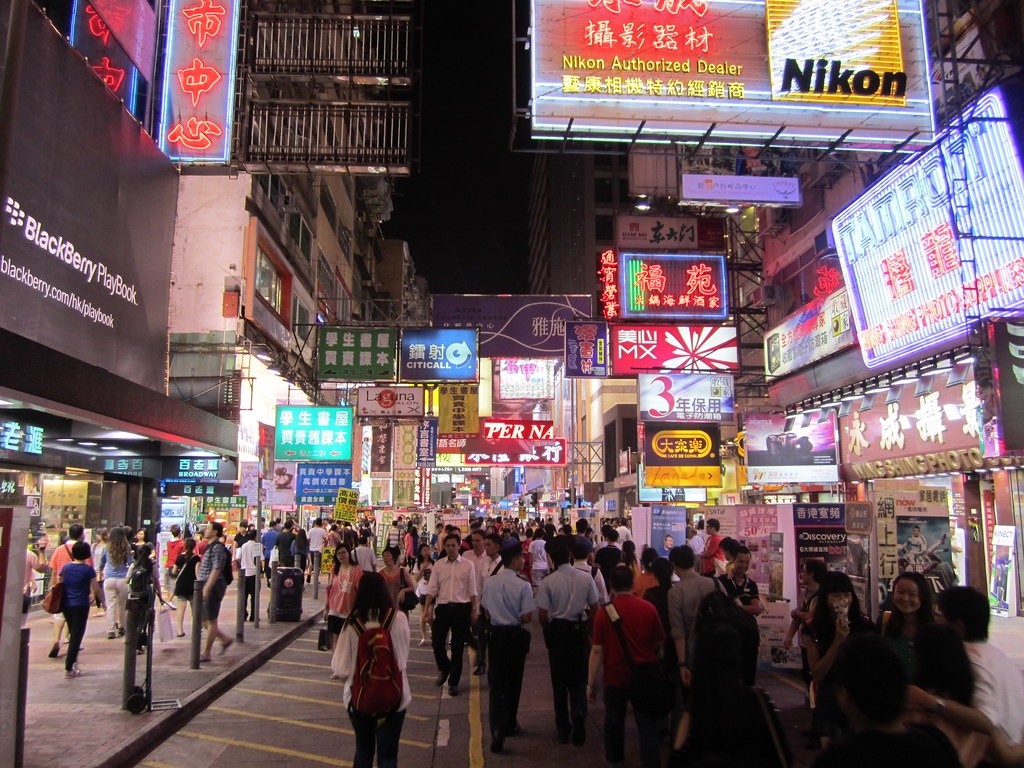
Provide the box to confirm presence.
[204,542,234,585]
[347,607,403,717]
[129,559,156,592]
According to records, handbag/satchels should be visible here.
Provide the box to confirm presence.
[318,620,332,651]
[172,583,177,594]
[399,567,419,612]
[400,555,407,567]
[157,605,173,642]
[43,582,64,614]
[628,662,676,718]
[694,578,738,630]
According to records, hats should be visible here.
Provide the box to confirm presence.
[498,539,521,556]
[545,535,574,554]
[572,535,593,553]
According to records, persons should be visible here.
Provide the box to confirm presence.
[324,502,793,768]
[96,527,135,640]
[901,523,927,563]
[126,544,164,654]
[59,541,106,677]
[779,560,1024,768]
[171,538,200,637]
[198,522,234,662]
[91,521,157,617]
[165,524,227,607]
[49,524,94,657]
[664,535,674,551]
[20,539,46,626]
[232,515,376,622]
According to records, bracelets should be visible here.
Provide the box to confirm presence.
[931,697,945,710]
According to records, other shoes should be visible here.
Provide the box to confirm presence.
[200,654,211,661]
[436,662,450,686]
[219,638,233,654]
[803,730,811,738]
[473,662,485,676]
[307,574,311,584]
[177,632,185,637]
[490,712,586,754]
[603,756,624,768]
[49,628,144,679]
[418,639,428,646]
[449,685,458,697]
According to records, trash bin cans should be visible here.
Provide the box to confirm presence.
[268,567,304,621]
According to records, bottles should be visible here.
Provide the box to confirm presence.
[761,540,769,573]
